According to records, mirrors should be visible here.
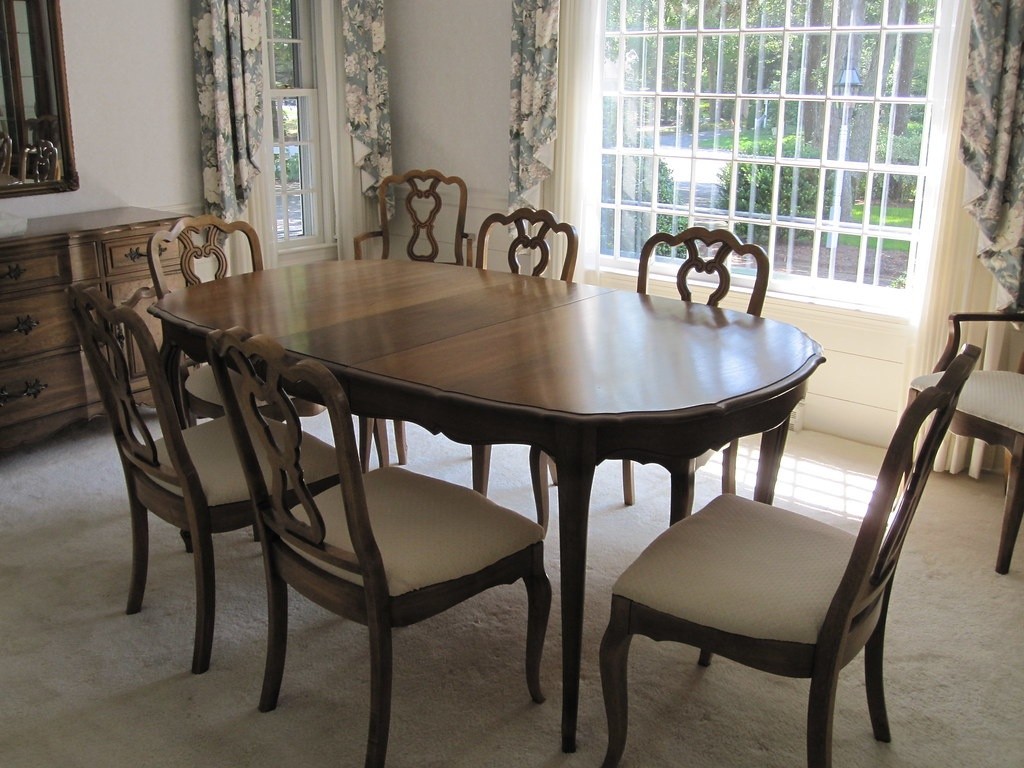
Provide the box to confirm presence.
[0,0,81,200]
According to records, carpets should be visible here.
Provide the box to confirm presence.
[0,401,1024,768]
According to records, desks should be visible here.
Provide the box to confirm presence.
[147,259,827,754]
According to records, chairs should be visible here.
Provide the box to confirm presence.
[20,139,60,184]
[0,132,13,176]
[207,326,553,768]
[600,344,983,768]
[62,283,340,675]
[147,215,325,423]
[353,168,770,540]
[902,312,1024,576]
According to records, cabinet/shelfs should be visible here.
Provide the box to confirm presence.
[0,206,195,451]
